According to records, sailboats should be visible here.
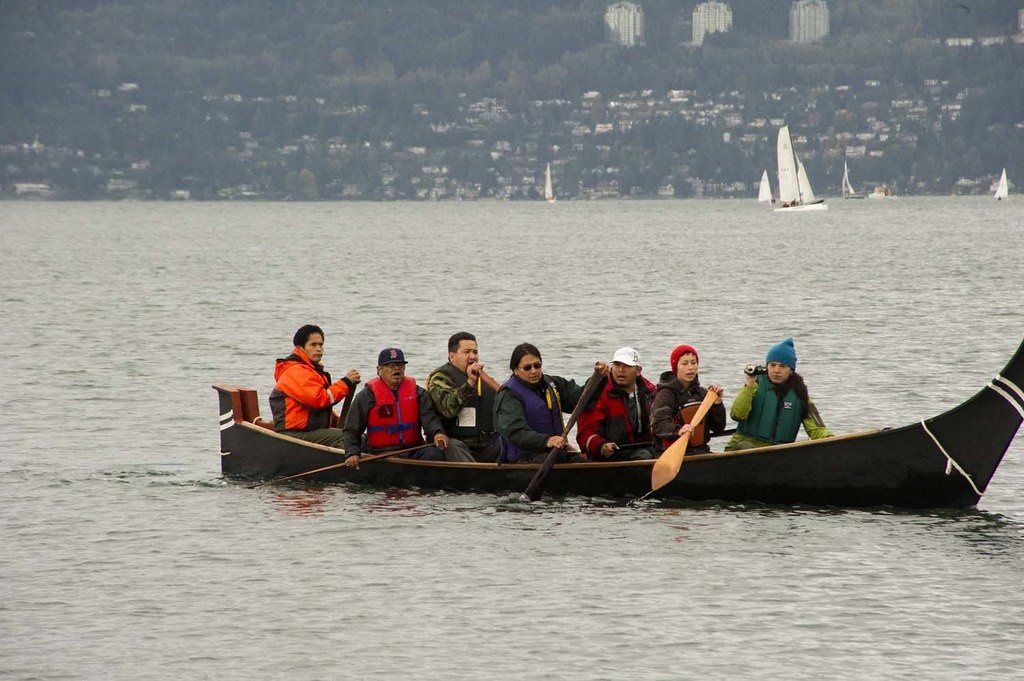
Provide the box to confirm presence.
[993,168,1009,200]
[757,169,777,206]
[544,160,557,203]
[771,124,829,213]
[841,161,866,199]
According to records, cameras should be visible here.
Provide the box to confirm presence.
[748,365,768,376]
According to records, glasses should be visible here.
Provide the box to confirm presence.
[517,360,541,371]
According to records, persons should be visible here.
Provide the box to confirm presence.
[649,345,727,458]
[576,347,658,463]
[268,324,366,452]
[341,348,448,471]
[724,338,835,453]
[426,332,501,462]
[495,343,609,470]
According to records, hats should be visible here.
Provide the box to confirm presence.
[670,345,699,376]
[766,337,796,372]
[609,346,641,368]
[378,349,408,366]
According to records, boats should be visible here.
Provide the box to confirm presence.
[211,337,1024,509]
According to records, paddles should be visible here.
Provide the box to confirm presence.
[246,439,445,489]
[471,366,501,392]
[519,362,605,502]
[652,385,722,492]
[338,382,357,429]
[612,428,738,451]
